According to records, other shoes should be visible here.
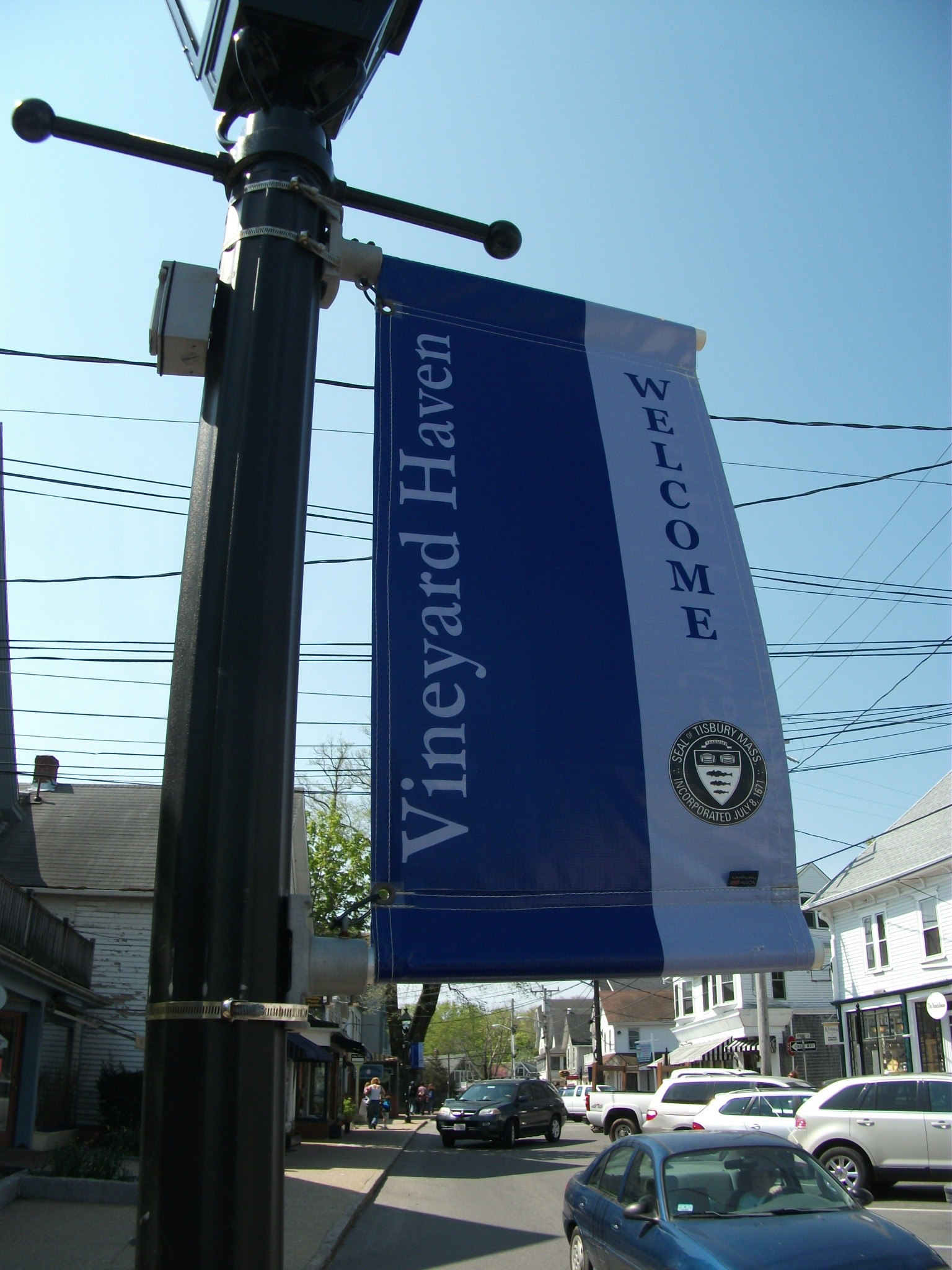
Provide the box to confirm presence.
[371,1123,376,1130]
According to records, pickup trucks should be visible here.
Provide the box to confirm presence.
[560,1084,618,1122]
[586,1069,760,1145]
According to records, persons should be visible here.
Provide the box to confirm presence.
[408,1080,435,1115]
[788,1071,798,1079]
[363,1077,392,1130]
[736,1164,784,1211]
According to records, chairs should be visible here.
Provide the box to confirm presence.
[614,1167,752,1211]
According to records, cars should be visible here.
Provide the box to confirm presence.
[563,1129,952,1270]
[641,1075,816,1134]
[692,1088,818,1139]
[787,1071,952,1203]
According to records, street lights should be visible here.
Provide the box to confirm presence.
[492,1024,516,1079]
[517,1016,552,1081]
[400,1006,411,1124]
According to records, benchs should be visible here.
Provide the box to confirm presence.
[859,1093,888,1110]
[752,1105,772,1116]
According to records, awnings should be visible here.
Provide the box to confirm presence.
[645,1036,779,1068]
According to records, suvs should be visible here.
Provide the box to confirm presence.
[436,1076,567,1149]
